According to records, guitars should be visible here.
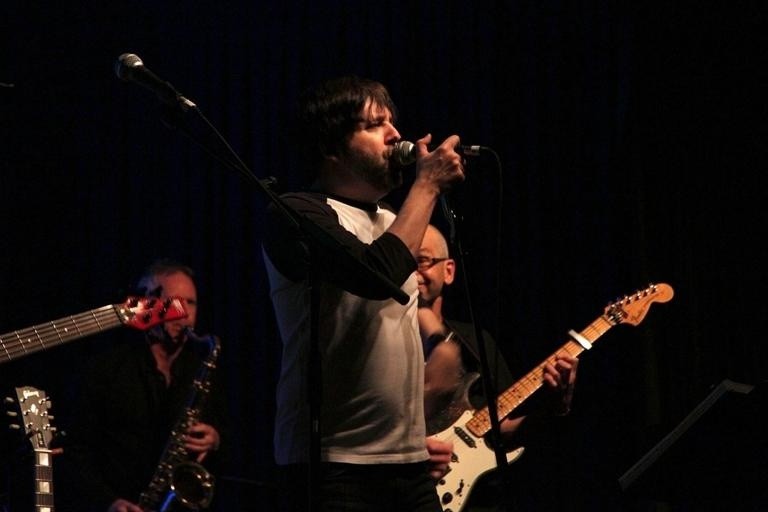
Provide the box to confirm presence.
[425,281,675,512]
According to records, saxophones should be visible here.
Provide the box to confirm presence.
[138,325,220,510]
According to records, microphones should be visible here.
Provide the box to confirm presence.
[114,53,196,116]
[397,136,492,167]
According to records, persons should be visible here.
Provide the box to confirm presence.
[417,223,579,480]
[64,260,225,512]
[262,77,466,512]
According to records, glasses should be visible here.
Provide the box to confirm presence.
[417,255,448,267]
